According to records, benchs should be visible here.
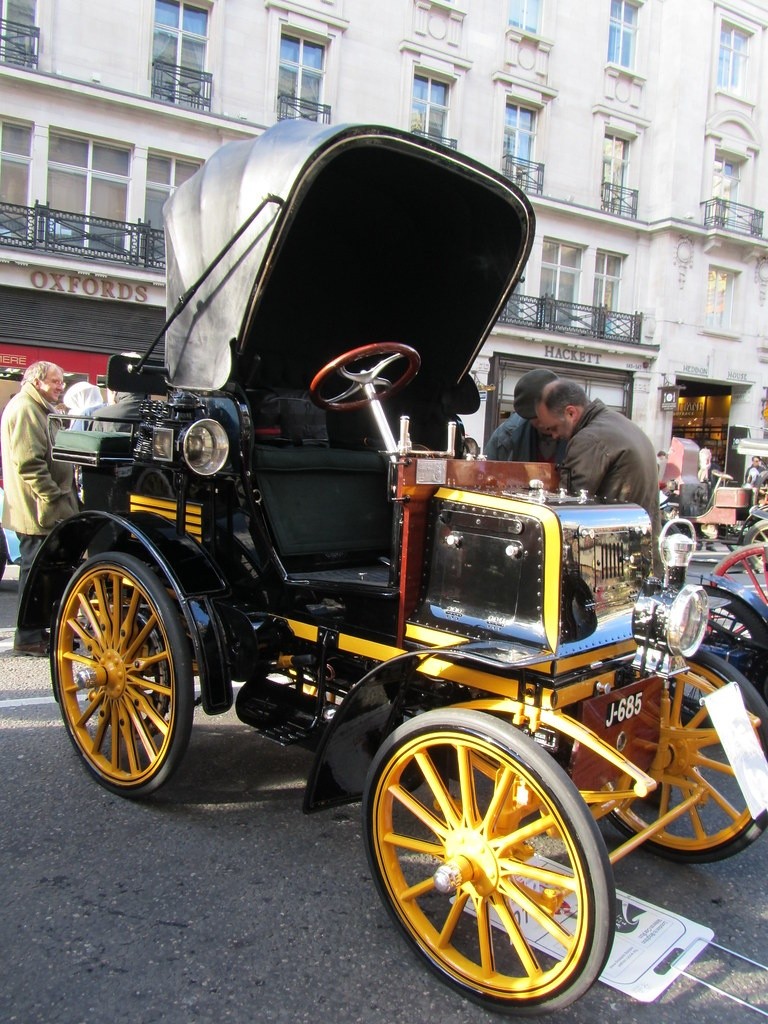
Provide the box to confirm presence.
[195,388,388,474]
[46,414,143,468]
[715,486,751,509]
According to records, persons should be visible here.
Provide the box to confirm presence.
[486,368,673,806]
[696,448,720,483]
[745,455,763,484]
[1,351,163,658]
[657,450,667,462]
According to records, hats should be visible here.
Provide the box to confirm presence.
[513,369,560,419]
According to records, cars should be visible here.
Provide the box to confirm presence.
[17,125,768,1019]
[650,436,768,572]
[640,513,768,743]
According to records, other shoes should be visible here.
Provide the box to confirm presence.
[13,632,81,658]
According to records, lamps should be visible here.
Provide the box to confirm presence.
[657,373,683,411]
